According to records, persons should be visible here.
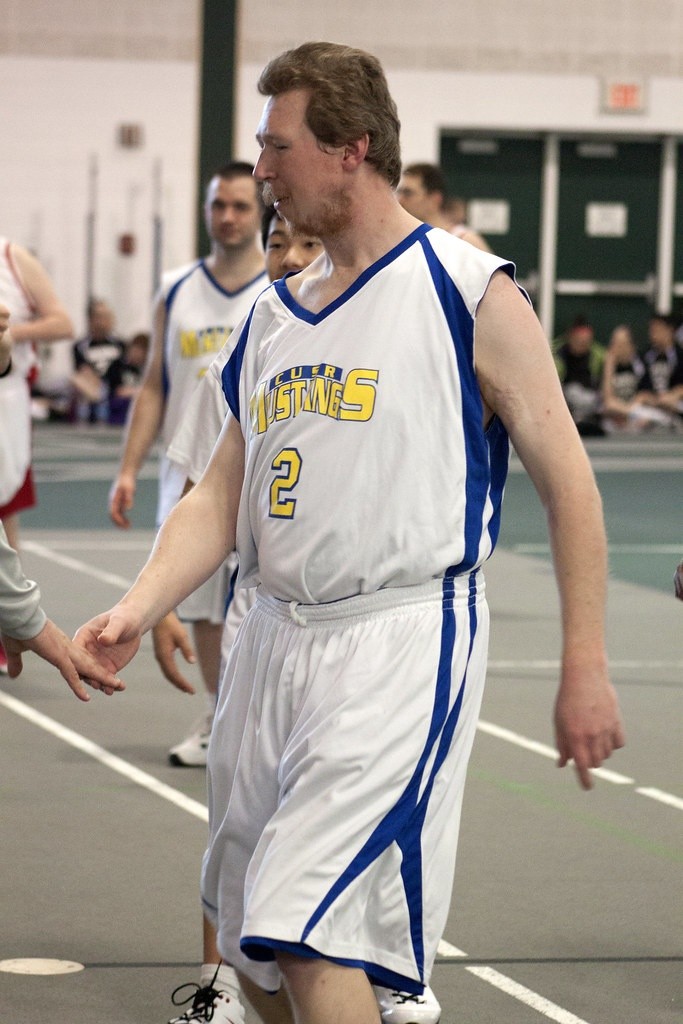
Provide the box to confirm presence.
[69,42,618,1023]
[1,164,683,1024]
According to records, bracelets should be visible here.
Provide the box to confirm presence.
[1,330,15,348]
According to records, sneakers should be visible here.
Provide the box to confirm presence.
[168,957,246,1024]
[167,720,212,767]
[372,985,443,1024]
